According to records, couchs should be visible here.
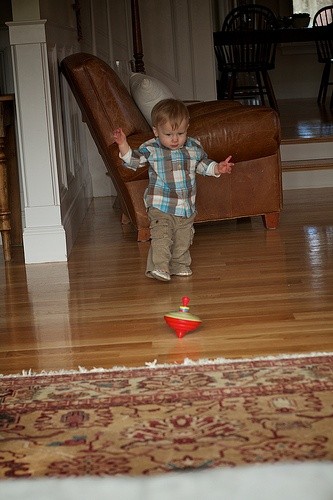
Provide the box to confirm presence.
[59,53,285,243]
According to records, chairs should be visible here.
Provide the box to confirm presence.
[213,2,281,118]
[312,4,333,111]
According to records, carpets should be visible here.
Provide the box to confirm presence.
[0,352,333,482]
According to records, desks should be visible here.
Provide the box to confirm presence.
[213,25,333,100]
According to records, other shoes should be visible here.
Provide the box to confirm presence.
[150,270,171,281]
[171,270,192,276]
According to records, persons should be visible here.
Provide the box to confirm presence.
[112,98,235,281]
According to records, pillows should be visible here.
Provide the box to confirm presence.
[128,71,179,130]
